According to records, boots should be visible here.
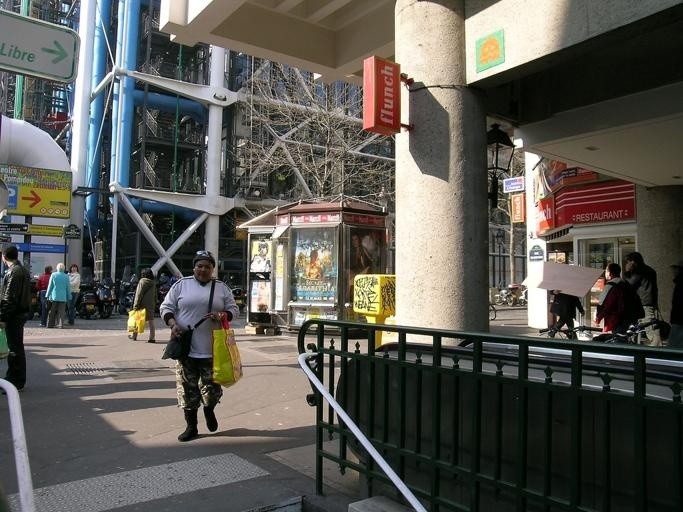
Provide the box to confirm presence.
[178,412,199,442]
[203,405,218,432]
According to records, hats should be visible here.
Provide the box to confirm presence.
[193,250,215,264]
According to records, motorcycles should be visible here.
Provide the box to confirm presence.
[519,289,527,306]
[21,264,247,319]
[493,282,518,308]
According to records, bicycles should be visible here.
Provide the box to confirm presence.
[592,318,659,345]
[537,319,603,342]
[488,301,497,321]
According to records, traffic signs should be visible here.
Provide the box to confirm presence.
[0,162,72,220]
[30,224,63,238]
[30,243,68,253]
[0,242,29,253]
[0,223,29,235]
[0,7,80,84]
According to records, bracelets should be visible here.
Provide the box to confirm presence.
[169,323,176,329]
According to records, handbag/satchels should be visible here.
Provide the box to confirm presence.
[163,329,192,359]
[213,329,243,386]
[127,309,147,333]
[653,319,670,341]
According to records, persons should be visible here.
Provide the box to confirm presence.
[157,248,240,443]
[618,252,666,347]
[548,289,585,339]
[349,232,372,273]
[43,262,71,329]
[0,247,32,394]
[127,267,156,344]
[593,262,645,345]
[35,265,55,328]
[251,242,271,272]
[64,263,81,326]
[669,264,683,347]
